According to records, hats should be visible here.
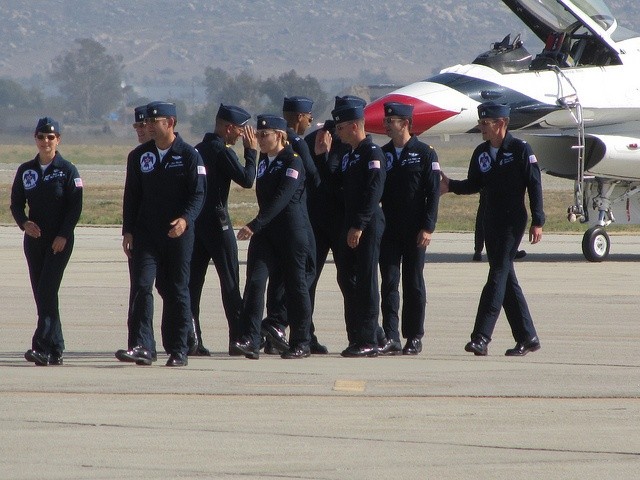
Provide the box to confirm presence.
[384,102,414,115]
[331,106,365,124]
[283,96,313,112]
[135,106,148,122]
[257,114,288,131]
[217,103,251,127]
[477,102,510,118]
[335,95,366,109]
[35,117,60,136]
[146,101,176,117]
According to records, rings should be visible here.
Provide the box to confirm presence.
[244,234,248,239]
[353,240,356,243]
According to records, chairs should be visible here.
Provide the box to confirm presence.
[529,51,574,70]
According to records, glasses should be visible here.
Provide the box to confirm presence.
[224,124,245,132]
[478,120,496,127]
[37,135,58,140]
[133,121,146,129]
[383,117,403,123]
[338,122,353,130]
[302,113,314,122]
[144,116,167,124]
[254,131,276,137]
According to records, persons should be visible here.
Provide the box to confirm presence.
[328,106,394,356]
[115,101,209,366]
[127,105,198,361]
[375,103,440,356]
[439,103,545,356]
[193,103,259,353]
[10,117,83,365]
[265,95,334,354]
[473,192,527,260]
[233,115,313,358]
[304,95,389,357]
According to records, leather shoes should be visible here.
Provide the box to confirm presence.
[281,344,310,359]
[514,251,526,259]
[231,338,259,359]
[197,345,210,356]
[309,342,328,354]
[505,334,540,356]
[402,337,422,355]
[378,338,387,347]
[49,351,63,365]
[377,338,402,356]
[151,350,157,361]
[187,316,200,356]
[465,338,487,356]
[165,352,188,366]
[340,343,378,357]
[229,337,267,356]
[115,344,152,365]
[264,341,283,355]
[261,316,289,350]
[473,255,481,261]
[25,350,48,366]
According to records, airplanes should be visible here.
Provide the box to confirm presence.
[364,0,640,262]
